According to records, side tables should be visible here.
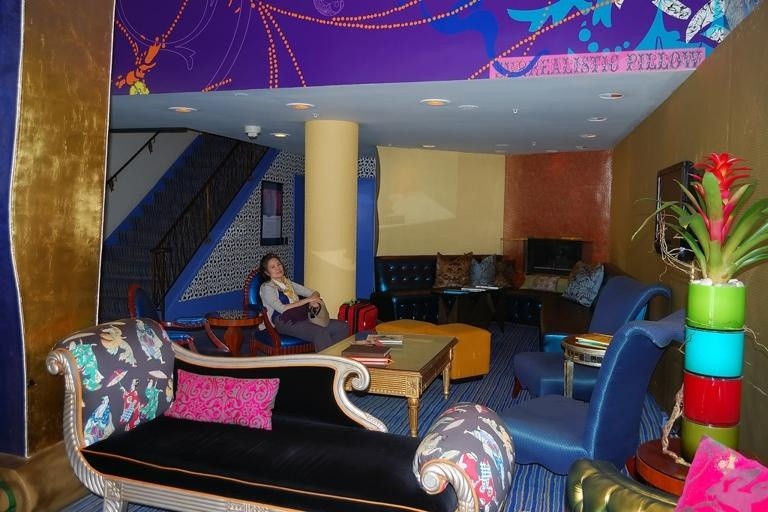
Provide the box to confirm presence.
[637,438,763,494]
[205,310,264,359]
[561,333,620,399]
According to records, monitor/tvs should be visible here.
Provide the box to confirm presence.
[655,161,703,260]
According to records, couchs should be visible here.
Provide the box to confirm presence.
[371,252,516,325]
[539,262,636,354]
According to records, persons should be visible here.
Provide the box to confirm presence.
[257,253,349,353]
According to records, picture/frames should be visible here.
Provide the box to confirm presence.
[260,180,284,246]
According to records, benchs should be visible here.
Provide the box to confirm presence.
[45,317,516,511]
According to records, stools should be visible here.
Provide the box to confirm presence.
[502,288,561,326]
[375,318,436,336]
[426,323,492,382]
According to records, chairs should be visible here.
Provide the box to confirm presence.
[512,275,671,403]
[243,268,316,355]
[127,283,235,358]
[564,459,678,512]
[497,309,687,475]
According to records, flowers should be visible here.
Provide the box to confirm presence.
[627,152,767,284]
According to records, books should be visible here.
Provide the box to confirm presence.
[461,287,488,292]
[341,334,404,365]
[476,285,503,290]
[574,332,613,350]
[442,288,469,294]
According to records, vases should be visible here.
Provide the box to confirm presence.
[680,280,746,463]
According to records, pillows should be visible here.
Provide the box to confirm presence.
[432,251,473,290]
[464,253,497,287]
[163,368,282,431]
[675,435,768,512]
[562,261,608,308]
[533,274,560,293]
[493,258,516,287]
